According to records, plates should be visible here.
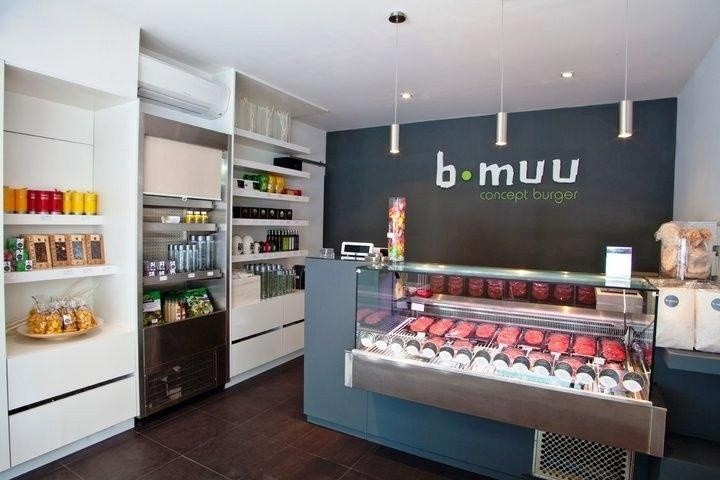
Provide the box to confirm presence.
[15,314,105,343]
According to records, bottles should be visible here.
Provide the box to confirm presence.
[266,229,300,251]
[185,210,209,224]
[168,234,216,273]
[244,262,297,299]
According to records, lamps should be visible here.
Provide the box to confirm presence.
[495,0,508,146]
[387,11,407,153]
[618,1,633,138]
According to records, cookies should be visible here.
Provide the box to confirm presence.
[27,307,97,335]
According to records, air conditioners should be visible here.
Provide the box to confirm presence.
[138,55,231,120]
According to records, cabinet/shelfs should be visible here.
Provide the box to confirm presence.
[232,67,328,390]
[136,101,232,420]
[3,58,140,479]
